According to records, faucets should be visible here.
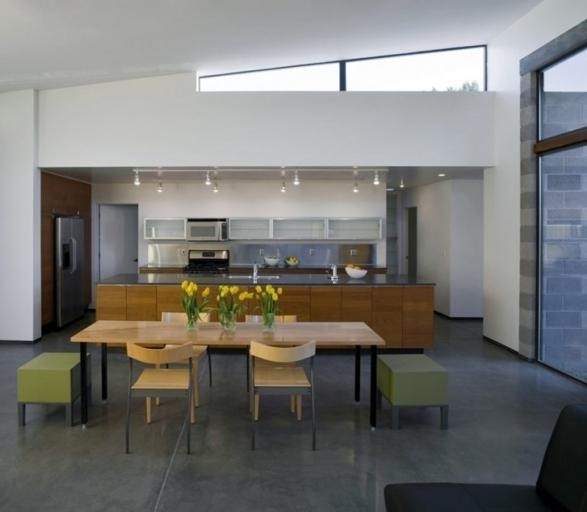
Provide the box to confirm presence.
[253,264,258,278]
[252,280,259,284]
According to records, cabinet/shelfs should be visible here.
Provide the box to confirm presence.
[228,216,384,242]
[211,283,249,318]
[183,280,213,317]
[142,217,187,242]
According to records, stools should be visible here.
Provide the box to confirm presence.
[14,350,93,429]
[375,349,452,434]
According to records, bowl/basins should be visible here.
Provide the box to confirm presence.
[343,267,367,279]
[264,258,279,267]
[285,260,299,266]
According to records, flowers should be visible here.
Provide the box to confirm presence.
[177,278,211,312]
[211,284,252,321]
[246,284,283,317]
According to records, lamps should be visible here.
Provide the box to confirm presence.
[129,167,382,197]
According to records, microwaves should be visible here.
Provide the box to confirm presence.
[186,218,228,242]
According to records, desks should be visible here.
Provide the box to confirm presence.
[71,319,382,434]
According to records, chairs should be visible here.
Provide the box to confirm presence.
[120,339,200,460]
[240,312,309,415]
[154,309,218,409]
[382,399,587,510]
[243,336,322,452]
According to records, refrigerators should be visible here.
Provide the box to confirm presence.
[52,216,112,327]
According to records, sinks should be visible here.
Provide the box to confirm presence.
[228,275,280,280]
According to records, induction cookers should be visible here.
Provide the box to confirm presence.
[184,249,230,272]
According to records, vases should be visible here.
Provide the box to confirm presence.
[263,309,276,329]
[186,314,198,327]
[221,312,238,335]
